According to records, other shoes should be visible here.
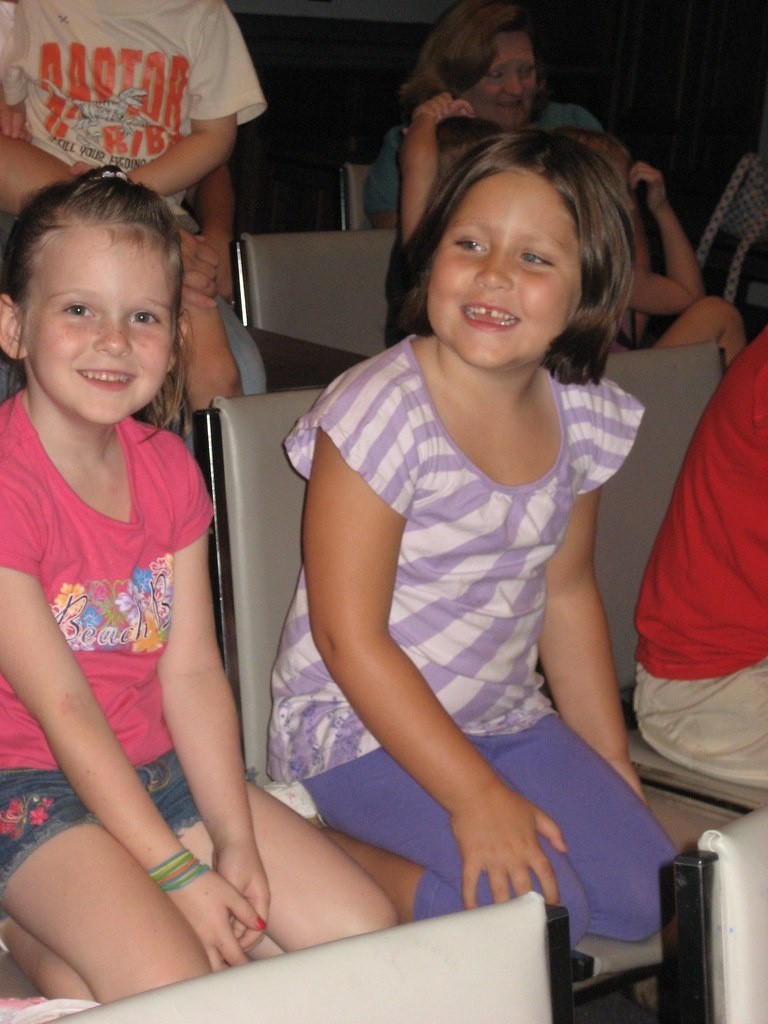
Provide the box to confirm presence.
[261,780,318,820]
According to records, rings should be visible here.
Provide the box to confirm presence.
[212,273,217,281]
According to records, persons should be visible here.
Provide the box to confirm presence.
[256,128,687,952]
[0,165,401,1001]
[630,322,767,789]
[362,1,749,373]
[0,0,243,470]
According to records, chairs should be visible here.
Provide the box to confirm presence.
[192,386,326,786]
[659,805,768,1024]
[29,891,553,1024]
[594,339,724,730]
[339,162,374,231]
[236,226,401,395]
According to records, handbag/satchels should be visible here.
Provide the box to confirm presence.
[696,153,768,305]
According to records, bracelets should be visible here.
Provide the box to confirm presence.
[144,847,210,892]
[652,198,669,215]
